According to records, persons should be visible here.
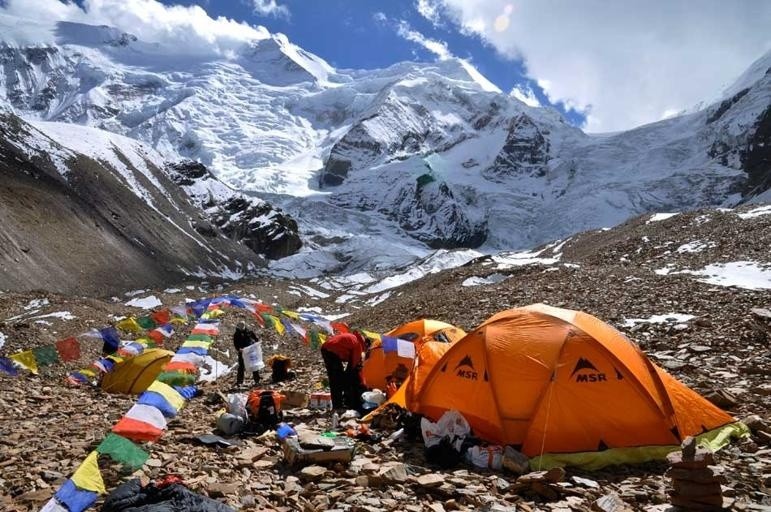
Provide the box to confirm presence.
[321,330,367,409]
[234,320,260,386]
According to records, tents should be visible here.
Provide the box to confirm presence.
[99,346,176,395]
[361,319,468,419]
[415,303,753,471]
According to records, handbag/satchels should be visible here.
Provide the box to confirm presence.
[246,390,286,424]
